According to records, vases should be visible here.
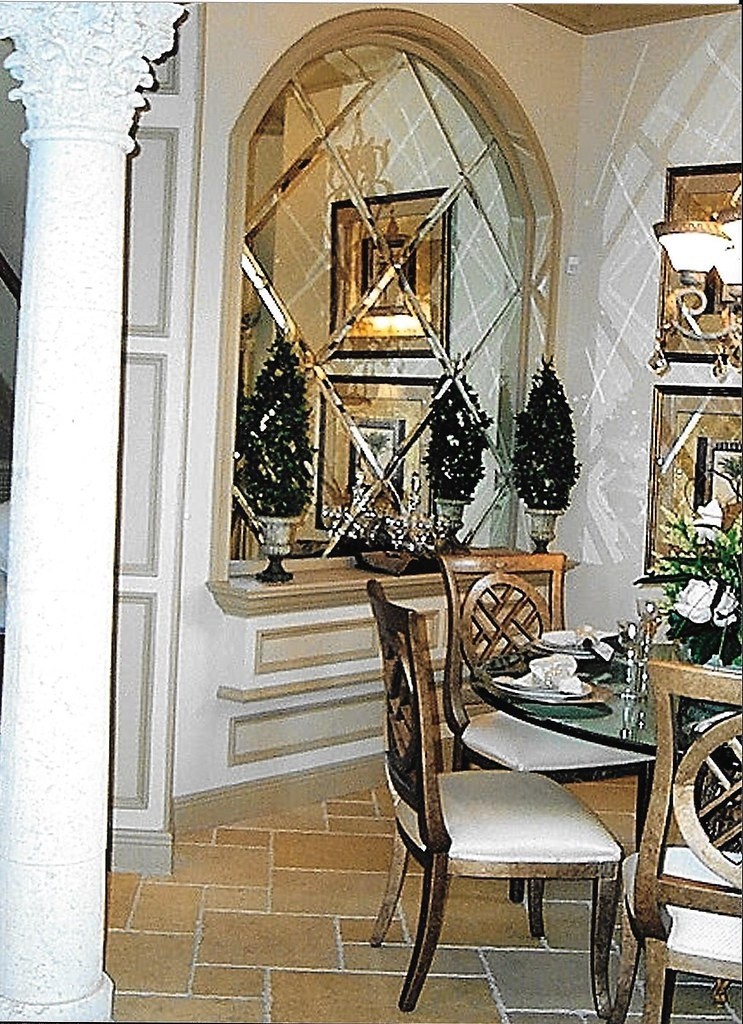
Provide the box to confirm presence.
[693,619,742,668]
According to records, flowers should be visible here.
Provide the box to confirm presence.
[632,458,743,662]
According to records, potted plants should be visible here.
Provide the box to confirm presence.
[244,326,320,585]
[421,360,496,548]
[511,354,581,552]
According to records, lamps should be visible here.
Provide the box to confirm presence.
[647,175,742,379]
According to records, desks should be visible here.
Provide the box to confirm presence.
[471,623,743,853]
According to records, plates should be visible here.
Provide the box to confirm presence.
[493,678,592,696]
[535,640,591,654]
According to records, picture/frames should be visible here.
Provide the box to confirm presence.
[314,374,438,531]
[644,383,743,576]
[657,162,742,364]
[327,187,452,359]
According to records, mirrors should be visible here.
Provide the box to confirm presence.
[227,38,533,560]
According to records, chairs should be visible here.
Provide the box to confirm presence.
[366,579,620,1020]
[613,660,743,1024]
[438,553,659,935]
